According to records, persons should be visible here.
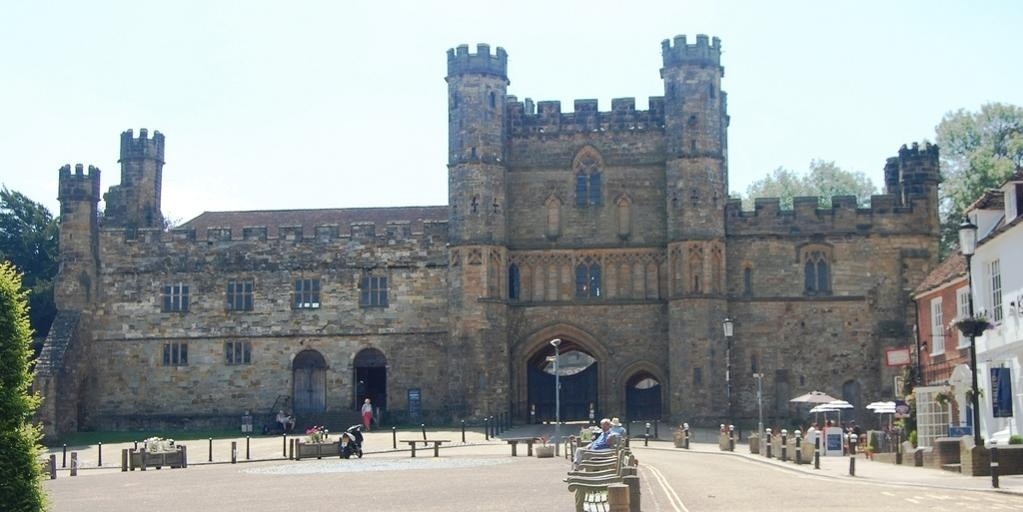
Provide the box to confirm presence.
[275,409,290,435]
[286,408,296,434]
[572,419,611,472]
[361,398,373,431]
[610,416,625,435]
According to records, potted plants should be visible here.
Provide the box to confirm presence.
[718,427,735,451]
[747,424,815,465]
[909,430,917,449]
[672,427,691,448]
[536,435,554,458]
[864,445,874,460]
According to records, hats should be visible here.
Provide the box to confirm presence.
[364,398,371,403]
[609,417,623,427]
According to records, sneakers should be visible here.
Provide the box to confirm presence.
[573,461,579,472]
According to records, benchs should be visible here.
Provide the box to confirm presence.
[562,434,639,512]
[501,437,540,456]
[399,439,452,456]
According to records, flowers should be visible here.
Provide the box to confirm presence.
[946,309,995,331]
[146,437,163,452]
[305,426,325,443]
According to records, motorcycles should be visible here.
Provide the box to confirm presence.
[337,424,362,458]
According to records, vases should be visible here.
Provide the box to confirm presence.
[295,437,342,460]
[42,455,56,479]
[956,320,990,337]
[129,445,187,471]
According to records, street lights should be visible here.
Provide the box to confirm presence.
[721,316,734,428]
[753,371,765,438]
[549,339,562,455]
[955,214,992,446]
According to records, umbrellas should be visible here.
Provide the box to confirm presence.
[789,389,901,433]
